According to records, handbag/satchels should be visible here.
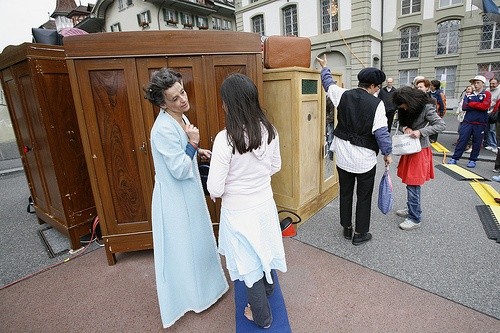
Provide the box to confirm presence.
[392,134,421,155]
[378,161,393,215]
[198,154,210,195]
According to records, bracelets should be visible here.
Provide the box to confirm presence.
[188,141,199,150]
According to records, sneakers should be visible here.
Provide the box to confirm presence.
[396,208,408,218]
[399,218,422,230]
[492,175,500,181]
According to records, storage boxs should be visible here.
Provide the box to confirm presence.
[263,33,311,69]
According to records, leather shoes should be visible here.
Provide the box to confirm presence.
[344,225,353,240]
[352,233,372,246]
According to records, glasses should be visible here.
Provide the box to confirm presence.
[377,84,382,89]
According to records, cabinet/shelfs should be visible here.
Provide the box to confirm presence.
[0,42,104,251]
[63,29,263,265]
[262,66,342,228]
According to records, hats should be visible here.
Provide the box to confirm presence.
[357,68,386,86]
[469,75,486,84]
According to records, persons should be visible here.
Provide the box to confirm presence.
[447,75,500,182]
[378,77,397,134]
[140,66,229,328]
[393,76,447,143]
[392,85,446,231]
[315,54,393,245]
[206,72,288,329]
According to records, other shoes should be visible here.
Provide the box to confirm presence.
[447,159,456,164]
[466,161,476,168]
